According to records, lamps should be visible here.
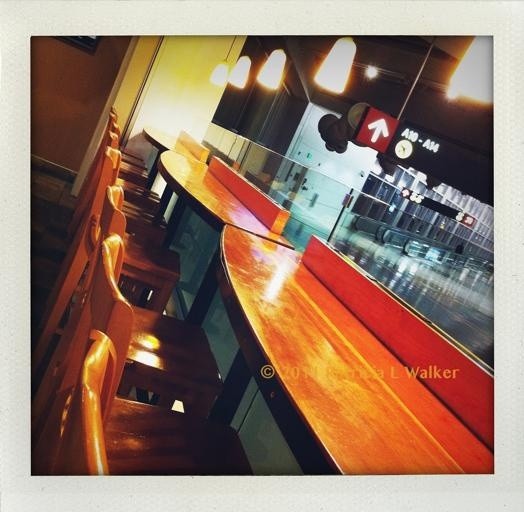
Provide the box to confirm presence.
[209,35,288,91]
[312,35,358,95]
[446,36,493,107]
